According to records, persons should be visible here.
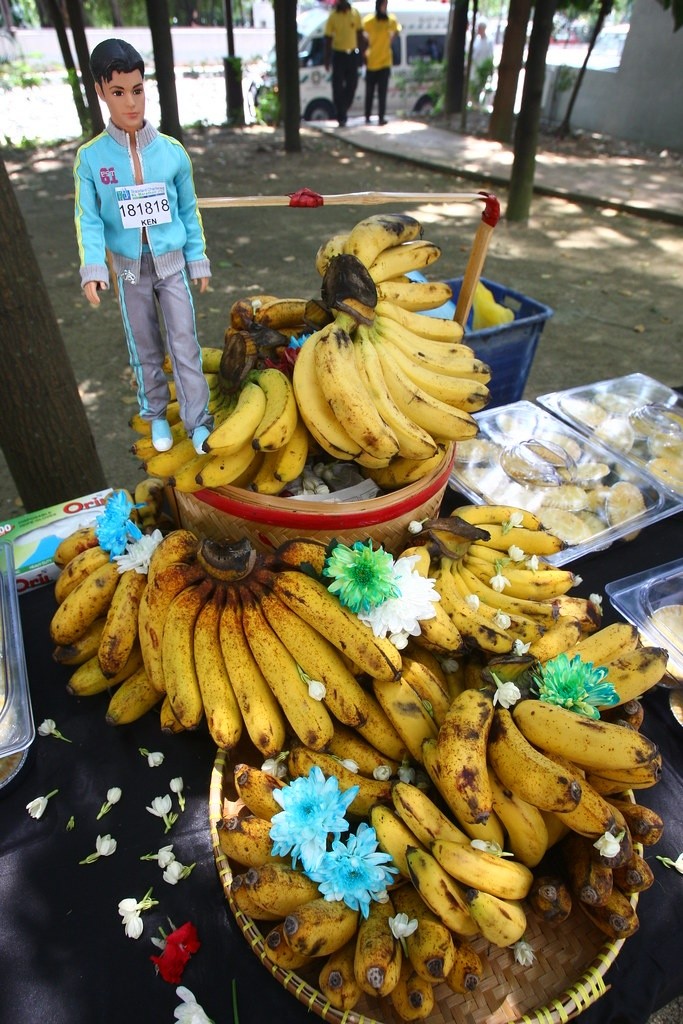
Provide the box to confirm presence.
[361,0,401,124]
[324,0,364,126]
[74,38,211,454]
[472,23,493,106]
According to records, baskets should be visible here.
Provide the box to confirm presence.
[208,676,645,1024]
[174,192,502,570]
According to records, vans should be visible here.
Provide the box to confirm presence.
[244,0,494,128]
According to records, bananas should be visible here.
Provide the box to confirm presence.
[214,503,671,1021]
[51,478,402,760]
[130,215,494,493]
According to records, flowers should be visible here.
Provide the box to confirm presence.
[23,492,626,1024]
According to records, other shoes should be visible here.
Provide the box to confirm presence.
[378,119,387,126]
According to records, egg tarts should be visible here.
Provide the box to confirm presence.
[449,393,683,552]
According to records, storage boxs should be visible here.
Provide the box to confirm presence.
[1,537,38,793]
[427,273,683,743]
